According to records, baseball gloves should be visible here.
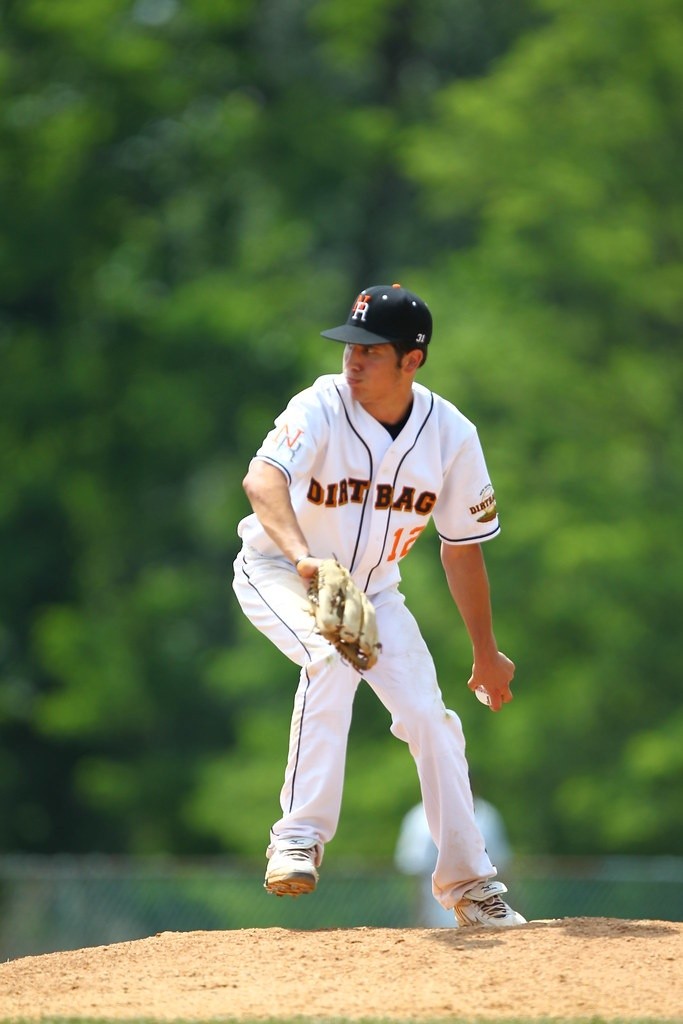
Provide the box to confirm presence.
[308,558,381,672]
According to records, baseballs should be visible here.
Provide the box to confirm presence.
[474,683,492,706]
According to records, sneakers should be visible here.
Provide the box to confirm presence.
[264,837,319,895]
[453,880,528,927]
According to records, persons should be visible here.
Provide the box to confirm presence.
[231,283,526,927]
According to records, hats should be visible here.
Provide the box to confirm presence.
[320,284,431,346]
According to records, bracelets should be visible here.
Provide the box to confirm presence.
[294,554,317,567]
